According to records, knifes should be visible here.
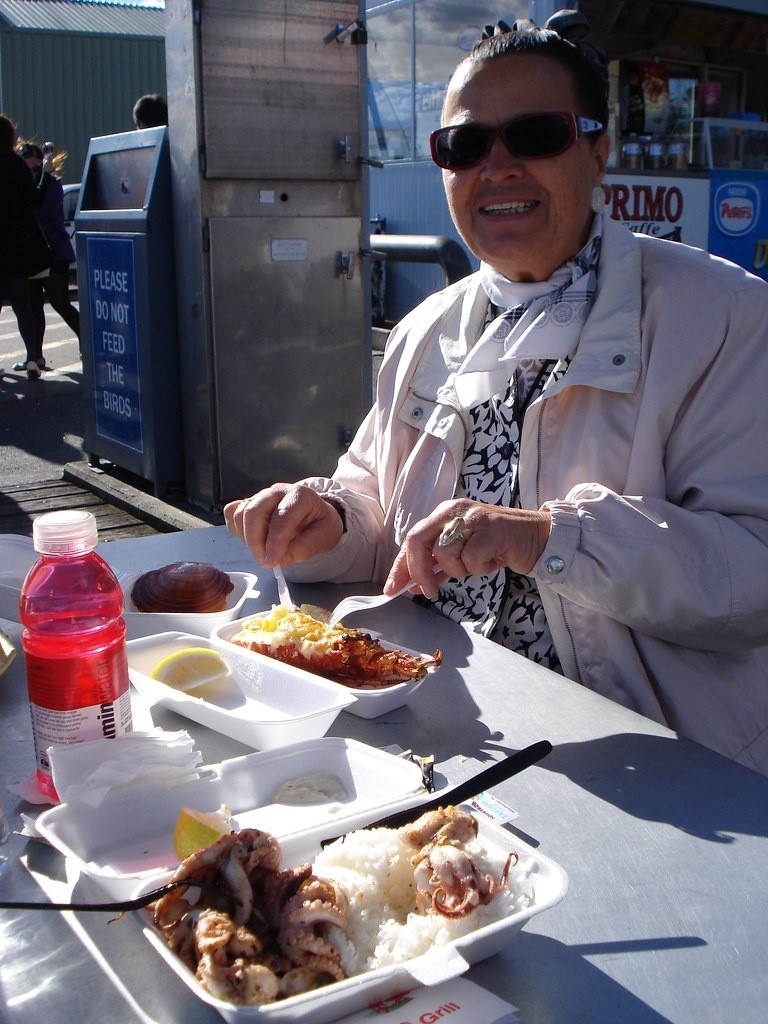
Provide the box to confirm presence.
[272,563,292,610]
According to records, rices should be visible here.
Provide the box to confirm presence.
[310,821,536,977]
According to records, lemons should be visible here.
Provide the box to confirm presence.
[152,647,229,691]
[174,803,232,861]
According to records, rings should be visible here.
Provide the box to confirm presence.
[438,516,464,547]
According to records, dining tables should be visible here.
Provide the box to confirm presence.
[0,528,768,1023]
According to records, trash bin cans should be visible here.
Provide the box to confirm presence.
[74,125,185,496]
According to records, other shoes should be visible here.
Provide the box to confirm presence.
[26,360,41,379]
[13,356,45,371]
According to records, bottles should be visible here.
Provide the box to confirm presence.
[18,510,133,802]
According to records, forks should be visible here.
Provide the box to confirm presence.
[323,563,443,636]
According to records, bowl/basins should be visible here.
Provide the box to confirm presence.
[34,735,571,1023]
[126,610,440,752]
[0,533,260,636]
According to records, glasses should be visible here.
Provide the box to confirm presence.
[429,111,603,172]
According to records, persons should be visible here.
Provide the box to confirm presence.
[0,117,42,380]
[12,142,79,370]
[224,30,768,775]
[133,95,168,129]
